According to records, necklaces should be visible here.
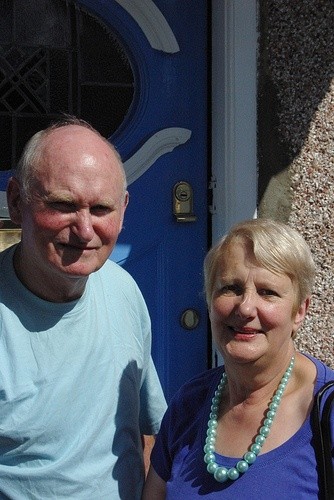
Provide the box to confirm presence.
[202,345,295,483]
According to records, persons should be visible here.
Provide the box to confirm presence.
[0,112,172,499]
[140,216,334,500]
